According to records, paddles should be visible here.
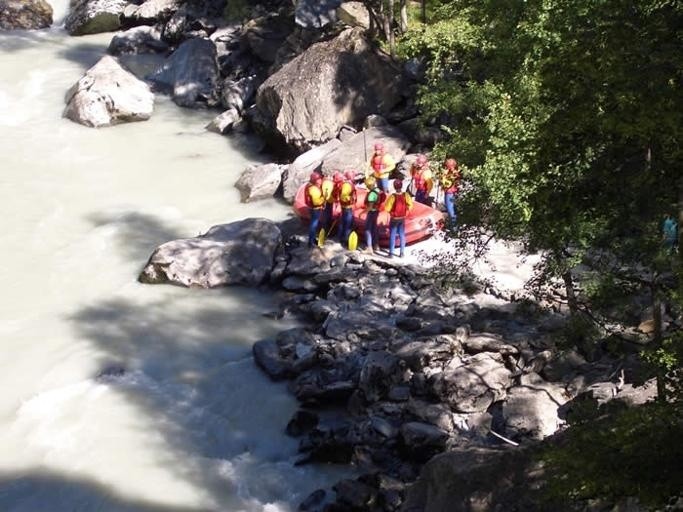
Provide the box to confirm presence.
[347,224,358,250]
[363,128,368,179]
[317,189,325,246]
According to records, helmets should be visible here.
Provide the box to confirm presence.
[310,172,322,183]
[364,177,376,188]
[416,154,427,166]
[445,158,456,169]
[332,172,343,183]
[374,142,385,152]
[345,171,355,181]
[394,179,402,189]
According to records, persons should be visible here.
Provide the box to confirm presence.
[301,143,463,257]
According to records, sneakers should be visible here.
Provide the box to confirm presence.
[308,240,405,259]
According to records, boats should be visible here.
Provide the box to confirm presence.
[293,181,446,247]
